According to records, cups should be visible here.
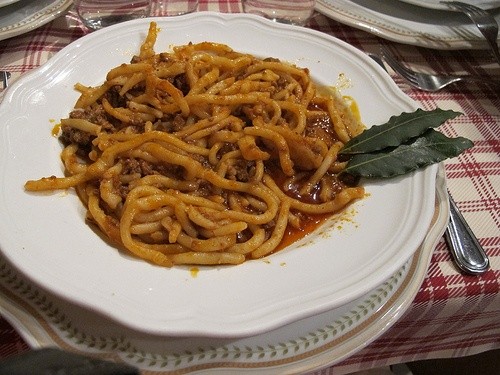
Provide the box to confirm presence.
[239,0,315,27]
[75,0,152,30]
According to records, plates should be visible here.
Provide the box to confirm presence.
[314,0,500,51]
[0,12,438,338]
[0,65,449,375]
[400,0,500,12]
[0,0,75,40]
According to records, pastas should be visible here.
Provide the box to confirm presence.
[23,21,370,266]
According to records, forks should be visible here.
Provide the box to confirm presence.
[440,1,500,66]
[378,43,500,92]
